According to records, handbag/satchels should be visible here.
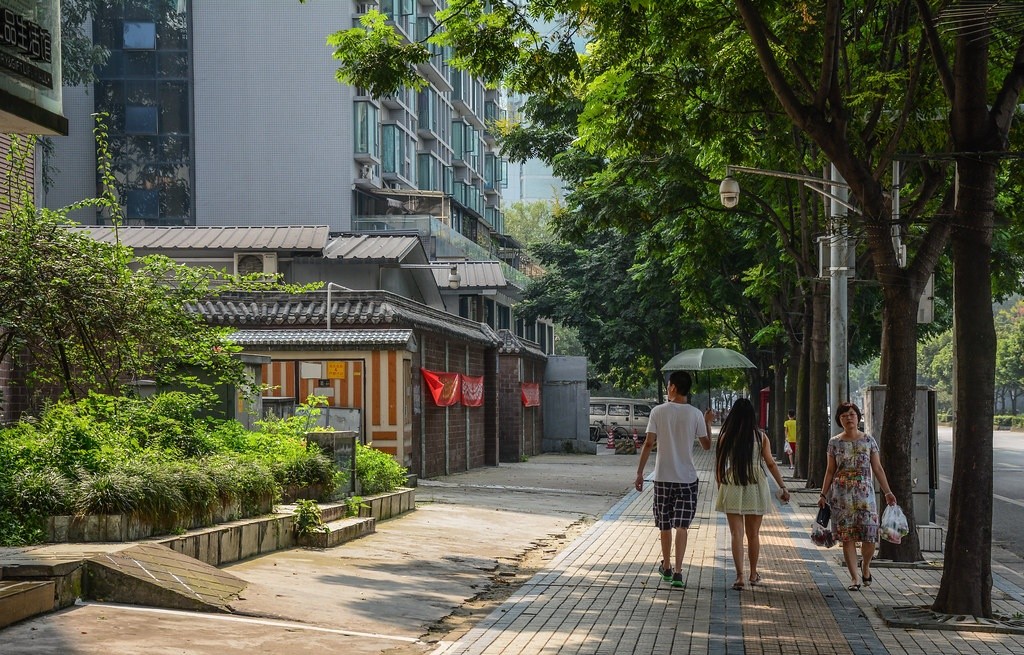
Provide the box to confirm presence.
[784,441,793,455]
[810,502,839,548]
[880,502,909,544]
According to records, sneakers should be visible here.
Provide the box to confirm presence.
[659,560,673,580]
[671,573,683,586]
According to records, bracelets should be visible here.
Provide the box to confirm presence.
[885,492,892,497]
[820,493,826,499]
[780,486,786,488]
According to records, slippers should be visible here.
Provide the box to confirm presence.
[860,560,872,586]
[848,585,860,590]
[751,573,761,585]
[789,466,793,469]
[730,582,743,590]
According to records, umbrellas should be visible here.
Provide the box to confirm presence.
[660,348,757,411]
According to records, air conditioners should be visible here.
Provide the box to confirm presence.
[234,252,277,283]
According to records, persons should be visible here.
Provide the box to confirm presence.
[784,409,796,470]
[635,370,714,591]
[714,398,790,591]
[716,408,720,421]
[817,402,896,592]
[720,408,725,426]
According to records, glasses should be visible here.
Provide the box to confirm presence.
[839,413,858,420]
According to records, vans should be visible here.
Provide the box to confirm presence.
[590,396,659,440]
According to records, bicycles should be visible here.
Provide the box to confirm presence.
[591,420,629,444]
